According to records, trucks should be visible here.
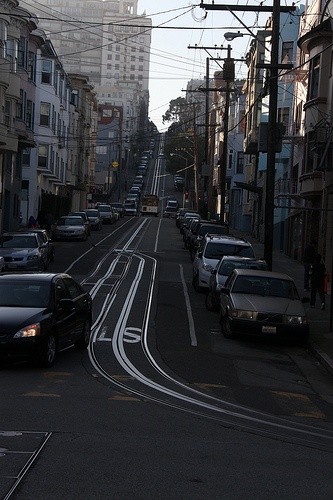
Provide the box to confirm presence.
[140,194,160,216]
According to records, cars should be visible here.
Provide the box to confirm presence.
[188,219,212,245]
[14,228,56,264]
[0,232,48,273]
[175,208,201,241]
[95,205,117,225]
[163,174,184,219]
[68,212,91,236]
[110,203,124,218]
[0,272,92,366]
[123,138,156,216]
[204,255,271,313]
[79,209,103,232]
[217,269,312,346]
[111,207,120,221]
[54,216,89,242]
[193,224,228,254]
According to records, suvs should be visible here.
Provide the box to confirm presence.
[192,234,256,293]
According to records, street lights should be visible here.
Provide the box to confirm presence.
[224,32,273,54]
